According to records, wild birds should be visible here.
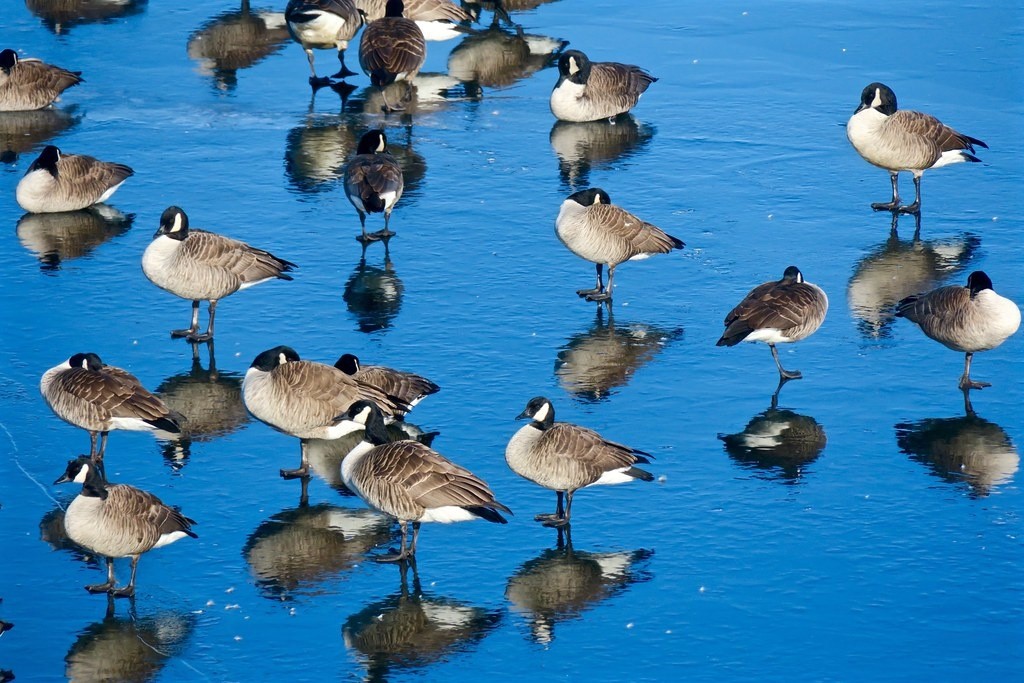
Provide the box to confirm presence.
[341,594,505,682]
[506,550,657,646]
[0,0,1023,601]
[63,593,203,683]
